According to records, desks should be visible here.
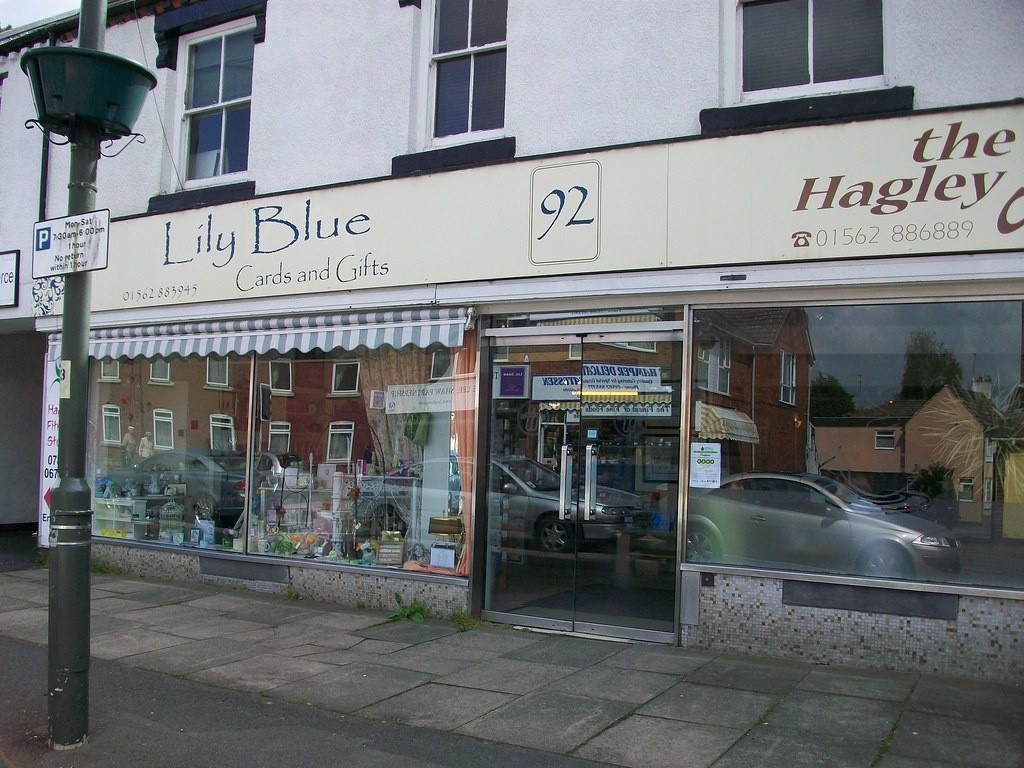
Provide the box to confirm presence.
[331,473,423,555]
[256,486,333,540]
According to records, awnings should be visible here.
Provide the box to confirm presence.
[699,404,760,446]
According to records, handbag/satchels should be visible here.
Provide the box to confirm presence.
[430,542,457,569]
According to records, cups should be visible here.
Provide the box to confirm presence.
[257,540,270,553]
[266,523,279,535]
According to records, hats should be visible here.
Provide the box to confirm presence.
[145,432,152,435]
[128,426,135,430]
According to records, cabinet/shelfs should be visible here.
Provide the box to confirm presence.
[118,493,192,539]
[92,497,146,539]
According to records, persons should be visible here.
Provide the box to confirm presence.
[138,431,155,459]
[362,442,372,464]
[122,426,136,466]
[572,453,615,488]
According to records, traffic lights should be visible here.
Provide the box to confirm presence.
[260,383,272,421]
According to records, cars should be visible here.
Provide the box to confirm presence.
[343,454,648,565]
[107,449,277,525]
[248,451,314,499]
[647,472,961,583]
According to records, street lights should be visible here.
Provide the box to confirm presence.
[19,45,158,744]
